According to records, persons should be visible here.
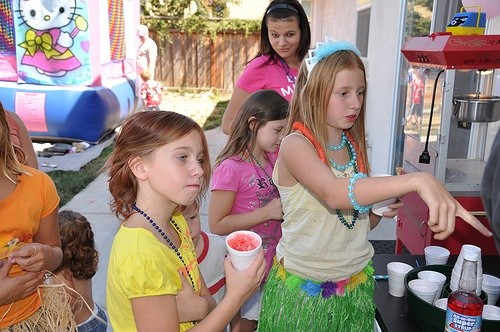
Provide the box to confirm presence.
[480,127,500,256]
[0,101,63,332]
[221,0,311,135]
[52,210,108,332]
[207,89,290,332]
[135,25,158,112]
[407,68,425,130]
[4,111,39,171]
[257,38,494,332]
[138,70,166,111]
[106,110,269,332]
[182,202,241,332]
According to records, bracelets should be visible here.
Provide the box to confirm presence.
[347,171,373,214]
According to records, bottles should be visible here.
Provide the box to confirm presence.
[444,253,483,332]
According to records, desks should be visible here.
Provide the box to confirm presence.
[372,254,500,332]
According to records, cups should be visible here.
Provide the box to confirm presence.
[387,262,413,297]
[450,244,482,296]
[482,305,500,320]
[417,270,446,306]
[424,246,451,266]
[482,274,500,306]
[435,298,448,310]
[371,174,397,216]
[408,279,438,305]
[225,230,262,272]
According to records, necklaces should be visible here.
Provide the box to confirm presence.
[326,132,359,229]
[132,206,198,325]
[245,146,280,198]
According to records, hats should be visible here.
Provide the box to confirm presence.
[137,25,148,37]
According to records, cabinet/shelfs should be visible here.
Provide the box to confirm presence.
[396,170,499,255]
